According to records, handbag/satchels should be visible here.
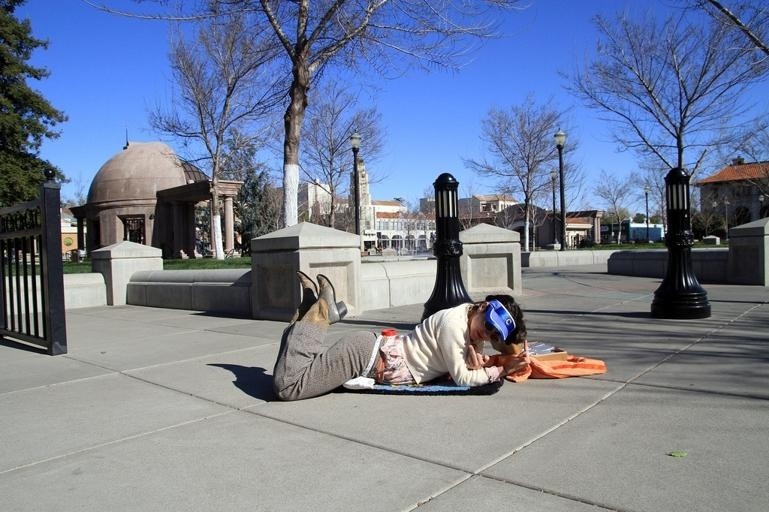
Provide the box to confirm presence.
[343,376,375,390]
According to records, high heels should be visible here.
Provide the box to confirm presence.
[296,270,347,326]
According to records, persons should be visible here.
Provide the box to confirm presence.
[272,270,531,401]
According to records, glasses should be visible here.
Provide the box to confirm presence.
[484,321,501,342]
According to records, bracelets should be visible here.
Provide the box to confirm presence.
[503,365,509,375]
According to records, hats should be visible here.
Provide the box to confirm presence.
[485,299,516,341]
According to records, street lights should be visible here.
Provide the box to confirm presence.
[644,186,651,241]
[758,194,765,219]
[350,130,362,235]
[550,127,567,251]
